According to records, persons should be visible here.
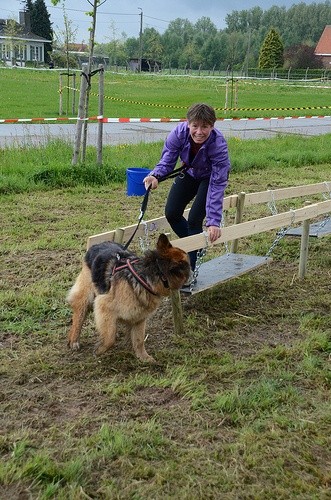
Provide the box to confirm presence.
[143,104,230,284]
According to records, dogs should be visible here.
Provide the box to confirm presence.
[65,233,192,363]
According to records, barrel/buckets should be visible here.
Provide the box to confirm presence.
[125,168,151,197]
